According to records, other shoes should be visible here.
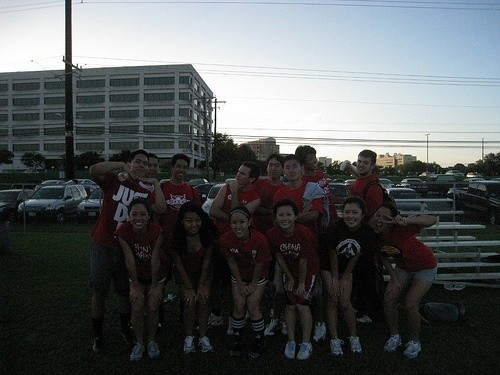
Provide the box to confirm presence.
[357,315,373,323]
[154,323,163,341]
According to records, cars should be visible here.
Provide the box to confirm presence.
[379,170,500,225]
[77,188,103,220]
[35,179,100,192]
[188,179,237,214]
[326,179,357,210]
[0,189,35,224]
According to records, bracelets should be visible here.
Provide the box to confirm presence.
[183,285,192,289]
[403,217,406,225]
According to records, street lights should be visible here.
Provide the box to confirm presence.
[426,133,430,163]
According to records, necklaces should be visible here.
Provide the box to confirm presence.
[284,231,293,234]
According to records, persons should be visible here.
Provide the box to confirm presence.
[90,149,167,352]
[116,198,163,361]
[267,198,318,361]
[216,206,272,359]
[319,196,376,359]
[150,153,201,305]
[197,161,262,335]
[171,202,218,354]
[370,203,437,358]
[263,145,337,342]
[145,153,160,224]
[343,150,382,216]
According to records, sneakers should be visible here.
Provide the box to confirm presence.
[227,316,234,335]
[330,337,345,356]
[311,320,327,342]
[384,333,402,354]
[346,335,362,354]
[122,330,137,346]
[230,333,242,357]
[197,336,215,357]
[403,341,421,359]
[284,340,297,359]
[129,343,145,362]
[197,312,224,330]
[146,341,160,359]
[263,318,279,337]
[296,341,313,361]
[249,339,266,358]
[279,319,288,335]
[92,335,106,350]
[182,335,196,353]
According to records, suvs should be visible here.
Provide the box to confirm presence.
[18,184,87,225]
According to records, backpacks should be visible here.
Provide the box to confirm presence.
[364,181,400,217]
